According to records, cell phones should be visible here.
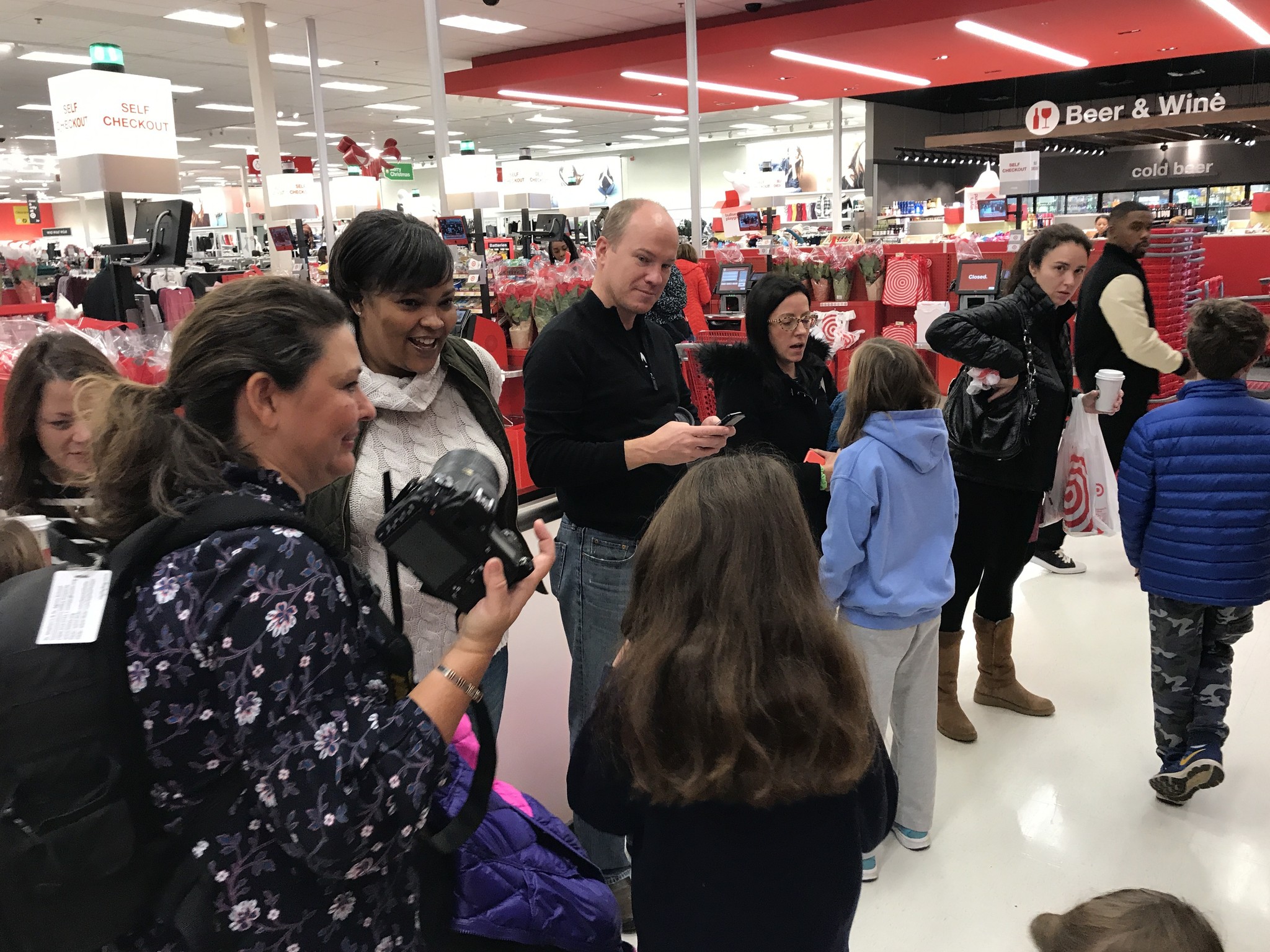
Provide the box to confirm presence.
[700,411,745,449]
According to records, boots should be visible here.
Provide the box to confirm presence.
[973,609,1056,716]
[937,629,977,741]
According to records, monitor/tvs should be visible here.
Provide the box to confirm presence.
[955,259,1002,294]
[737,211,763,231]
[716,263,753,293]
[977,195,1008,221]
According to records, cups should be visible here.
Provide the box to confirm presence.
[1094,369,1125,412]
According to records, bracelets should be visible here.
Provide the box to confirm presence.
[435,663,484,703]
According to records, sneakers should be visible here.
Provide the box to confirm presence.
[1155,765,1188,806]
[1029,548,1085,574]
[1150,744,1223,801]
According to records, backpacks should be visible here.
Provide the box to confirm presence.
[0,491,355,952]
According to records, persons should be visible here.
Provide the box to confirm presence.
[1121,300,1270,807]
[0,198,1194,952]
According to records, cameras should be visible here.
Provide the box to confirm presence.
[375,449,534,614]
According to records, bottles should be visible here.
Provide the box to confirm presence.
[1148,202,1193,220]
[1188,193,1199,205]
[881,201,924,216]
[872,224,903,236]
[836,236,850,242]
[1028,213,1055,229]
[882,238,897,244]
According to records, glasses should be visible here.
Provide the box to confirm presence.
[768,314,818,332]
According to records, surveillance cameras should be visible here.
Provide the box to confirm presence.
[0,138,6,142]
[745,3,762,13]
[482,0,500,6]
[605,143,612,146]
[428,156,434,159]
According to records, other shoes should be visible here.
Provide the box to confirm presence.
[608,876,636,932]
[891,820,932,850]
[862,856,878,880]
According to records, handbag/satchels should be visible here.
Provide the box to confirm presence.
[936,298,1036,461]
[1036,394,1122,537]
[882,253,932,364]
